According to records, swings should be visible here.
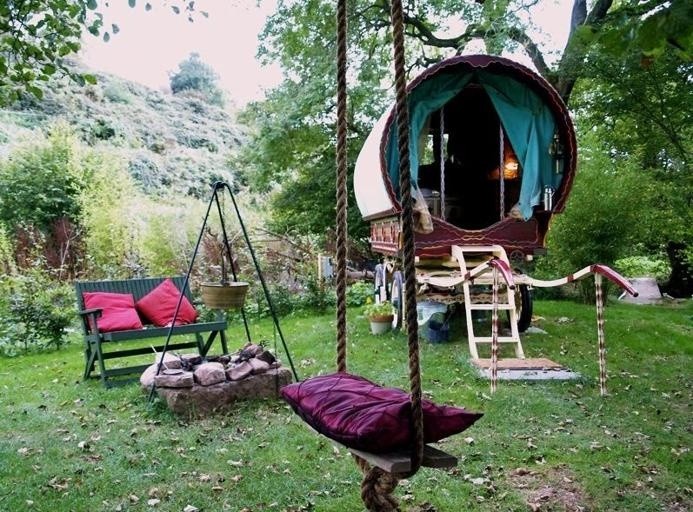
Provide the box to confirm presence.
[279,0,483,473]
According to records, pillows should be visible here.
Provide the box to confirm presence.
[135,279,198,327]
[83,292,143,333]
[278,371,485,447]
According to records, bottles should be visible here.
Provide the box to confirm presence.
[543,185,553,211]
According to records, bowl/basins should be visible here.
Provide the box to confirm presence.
[427,321,448,342]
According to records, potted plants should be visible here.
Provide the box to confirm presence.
[360,301,394,336]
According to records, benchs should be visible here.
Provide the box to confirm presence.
[75,276,228,390]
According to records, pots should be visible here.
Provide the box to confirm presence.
[199,256,248,310]
